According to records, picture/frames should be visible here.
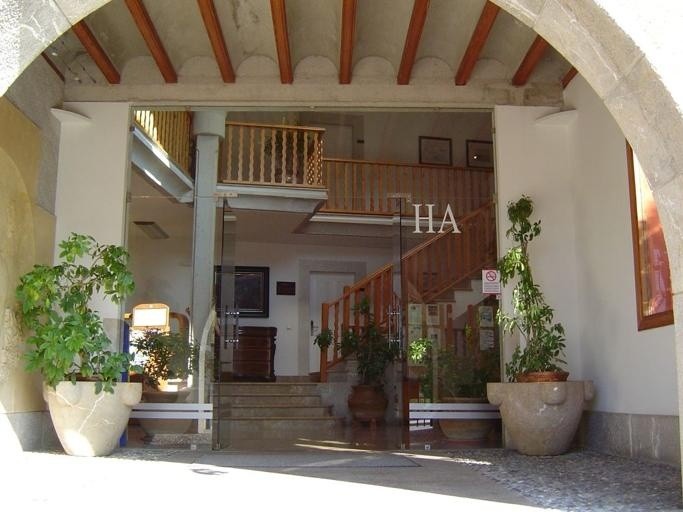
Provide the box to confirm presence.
[626,137,675,331]
[419,135,452,166]
[466,138,494,168]
[214,265,269,318]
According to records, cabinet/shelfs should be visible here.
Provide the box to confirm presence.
[216,325,277,382]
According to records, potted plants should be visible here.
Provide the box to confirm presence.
[477,198,570,382]
[409,321,499,440]
[264,130,309,183]
[16,229,142,457]
[130,328,212,439]
[313,301,400,427]
